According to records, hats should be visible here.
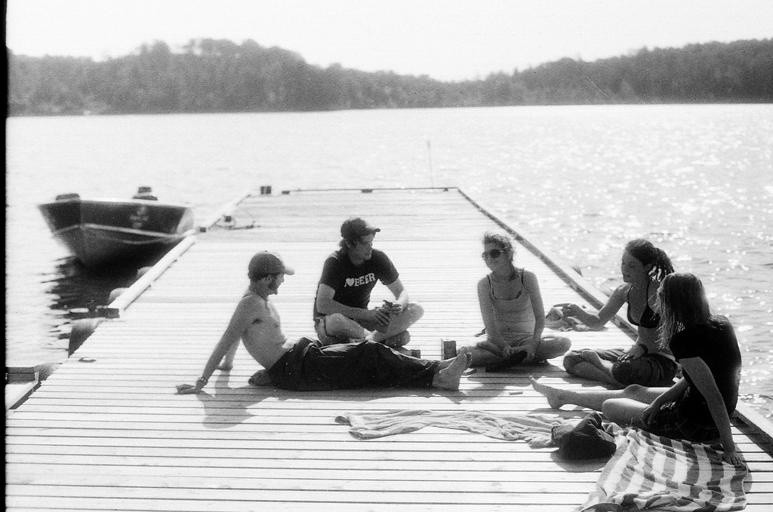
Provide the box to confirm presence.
[248,250,295,275]
[341,216,380,242]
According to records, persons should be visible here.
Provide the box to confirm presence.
[174,251,473,395]
[552,240,683,385]
[465,233,570,372]
[313,218,425,349]
[528,273,744,469]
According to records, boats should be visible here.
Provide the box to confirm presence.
[5,362,52,411]
[36,186,196,276]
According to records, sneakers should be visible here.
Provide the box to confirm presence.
[386,331,411,349]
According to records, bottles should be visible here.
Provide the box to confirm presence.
[376,299,392,334]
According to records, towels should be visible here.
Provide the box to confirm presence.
[572,426,748,512]
[336,407,590,447]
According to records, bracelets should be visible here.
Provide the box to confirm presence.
[199,376,208,384]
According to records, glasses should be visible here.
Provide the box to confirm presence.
[481,249,504,260]
